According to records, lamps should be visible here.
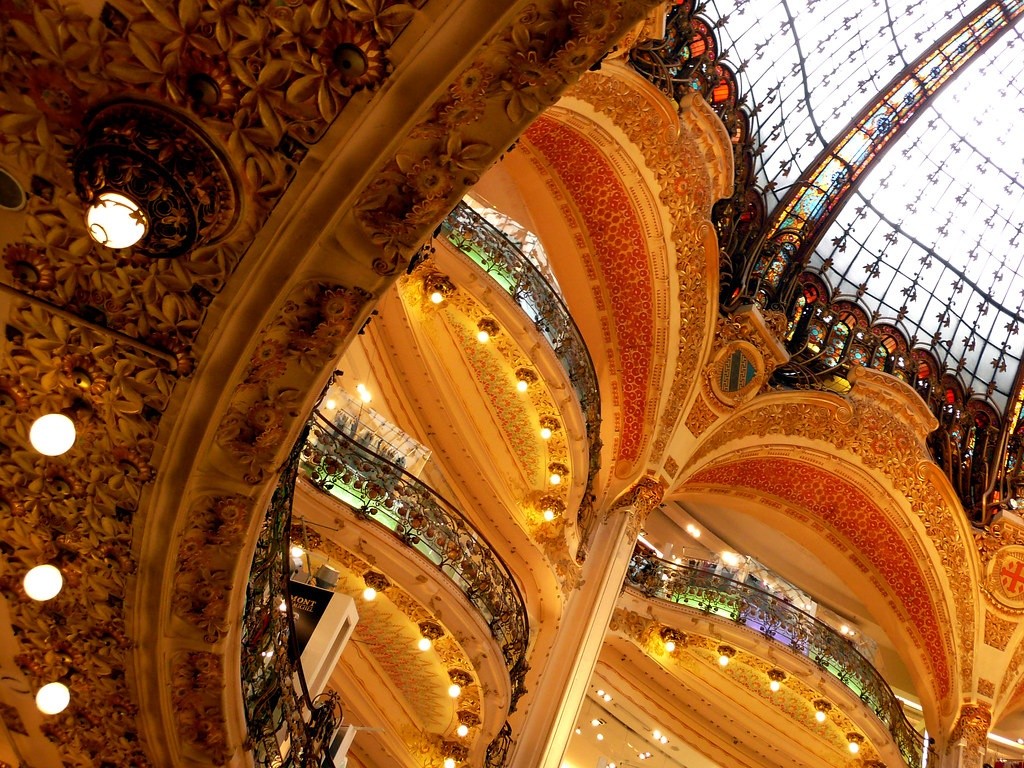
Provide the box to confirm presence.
[68,98,242,262]
